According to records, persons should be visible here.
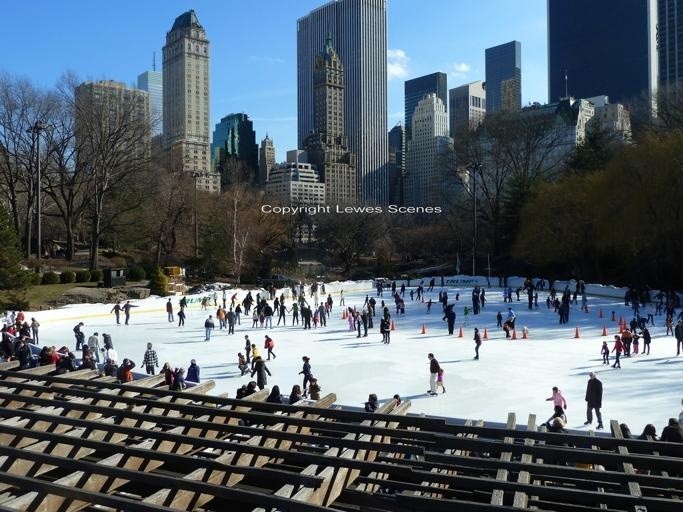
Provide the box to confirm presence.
[0,276,683,475]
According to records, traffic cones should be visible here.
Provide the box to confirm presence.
[421,324,425,334]
[599,309,625,336]
[342,305,356,319]
[522,327,526,338]
[512,329,516,339]
[575,327,578,338]
[458,325,462,337]
[483,328,486,338]
[390,320,395,330]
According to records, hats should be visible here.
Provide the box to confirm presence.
[190,358,196,364]
[553,417,565,427]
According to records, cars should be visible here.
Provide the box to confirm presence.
[237,273,262,287]
[264,275,301,290]
[215,274,237,284]
